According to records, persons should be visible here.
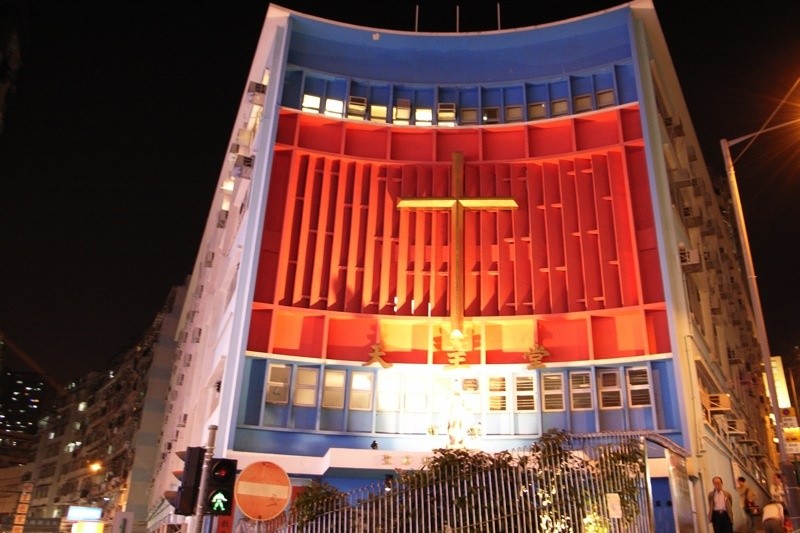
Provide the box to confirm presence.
[707,476,734,533]
[762,500,785,533]
[734,477,756,528]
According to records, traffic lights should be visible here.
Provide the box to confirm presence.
[206,458,238,516]
[164,446,203,516]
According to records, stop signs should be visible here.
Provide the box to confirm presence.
[234,461,291,520]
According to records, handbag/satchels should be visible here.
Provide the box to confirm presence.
[744,500,755,513]
